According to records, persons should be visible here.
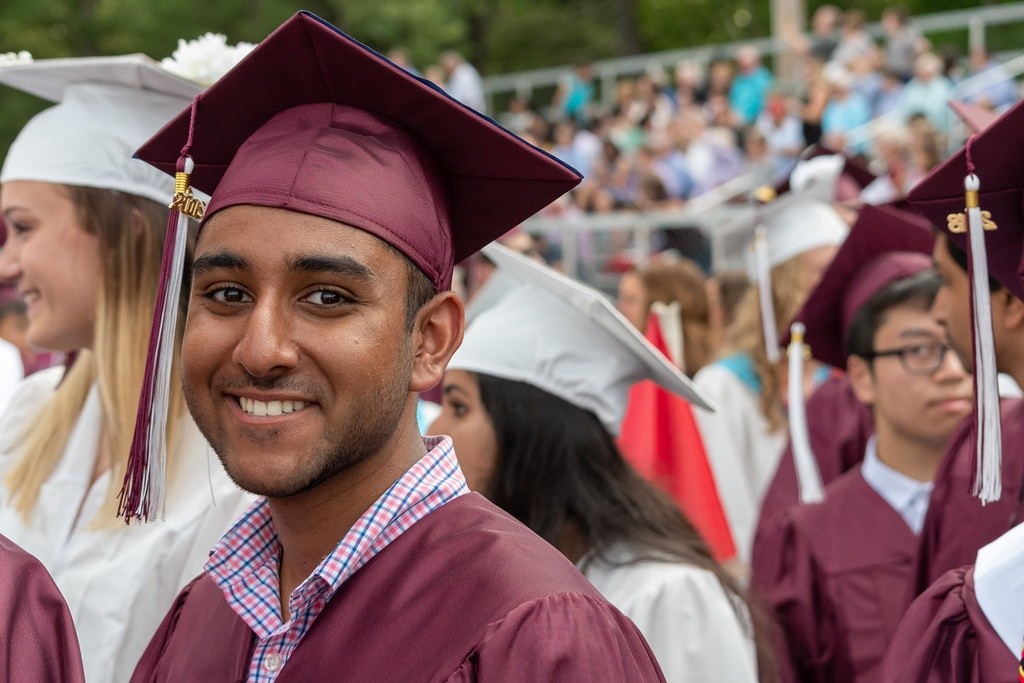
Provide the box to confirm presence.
[1,7,1024,683]
[128,104,667,683]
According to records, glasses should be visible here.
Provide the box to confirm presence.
[866,343,949,374]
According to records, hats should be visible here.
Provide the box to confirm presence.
[1,50,210,225]
[441,240,717,436]
[117,10,584,526]
[780,202,938,504]
[715,179,852,367]
[905,98,1024,507]
